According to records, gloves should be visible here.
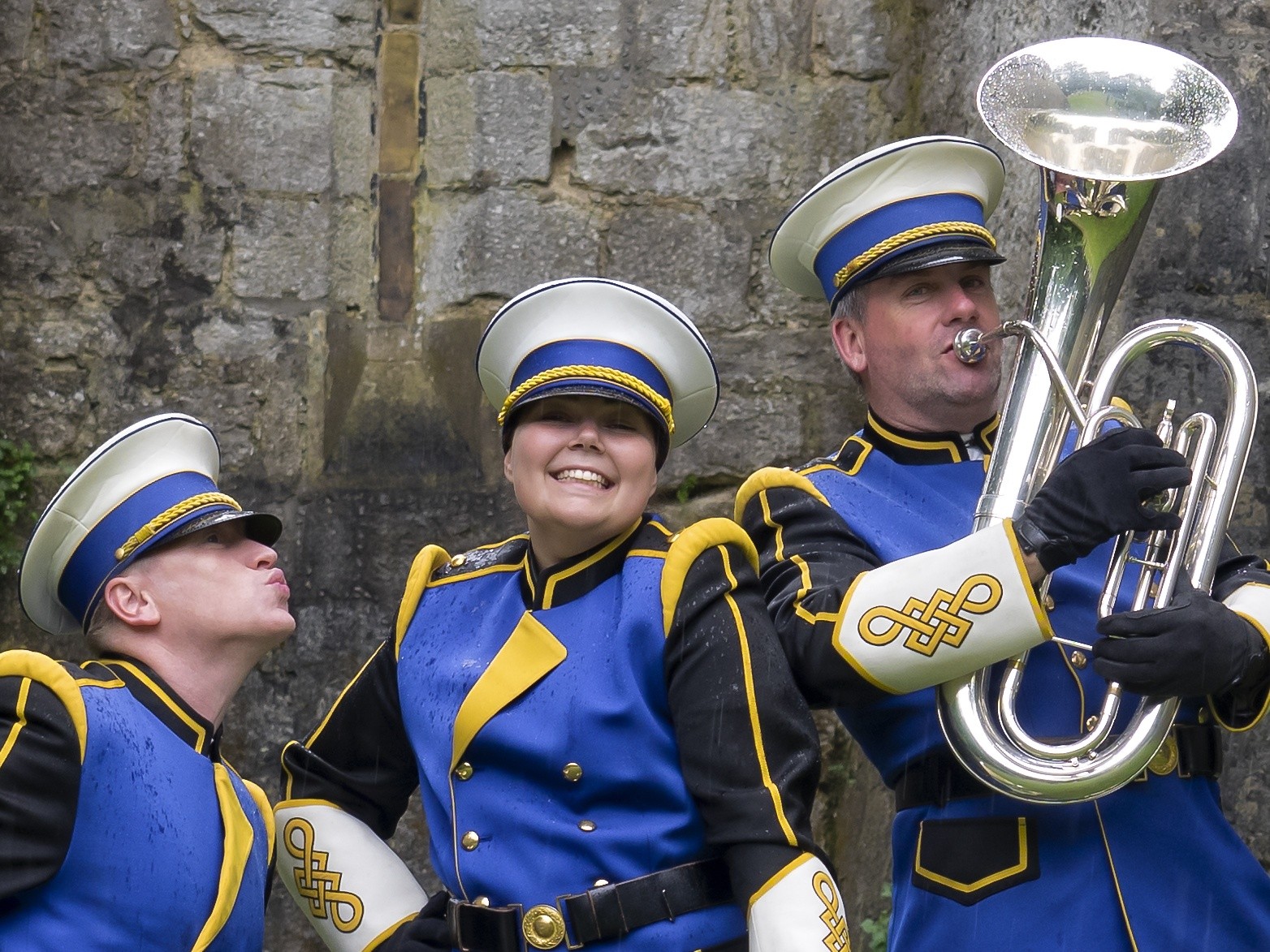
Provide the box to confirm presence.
[1090,538,1261,699]
[1012,425,1195,576]
[375,891,464,952]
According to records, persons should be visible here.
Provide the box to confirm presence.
[734,135,1269,952]
[271,278,853,952]
[0,412,298,952]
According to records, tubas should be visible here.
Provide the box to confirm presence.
[933,36,1259,805]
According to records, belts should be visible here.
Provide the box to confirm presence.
[443,855,735,952]
[892,722,1228,812]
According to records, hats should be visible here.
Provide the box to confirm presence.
[18,413,281,636]
[769,135,1005,316]
[475,275,721,467]
